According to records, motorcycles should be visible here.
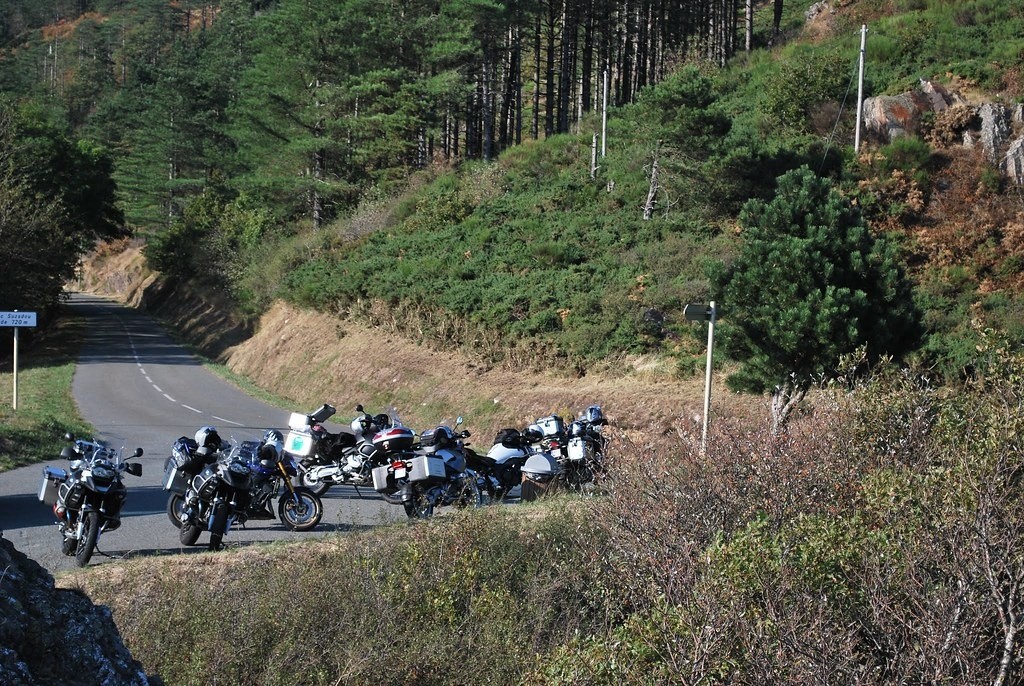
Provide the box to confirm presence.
[462,405,615,504]
[162,426,323,552]
[37,432,144,568]
[283,404,416,504]
[371,416,481,520]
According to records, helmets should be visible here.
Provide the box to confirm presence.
[261,441,285,463]
[195,426,222,452]
[527,424,544,443]
[351,416,370,434]
[434,426,453,444]
[586,406,603,423]
[568,421,586,437]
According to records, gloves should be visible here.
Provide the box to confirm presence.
[174,435,199,455]
